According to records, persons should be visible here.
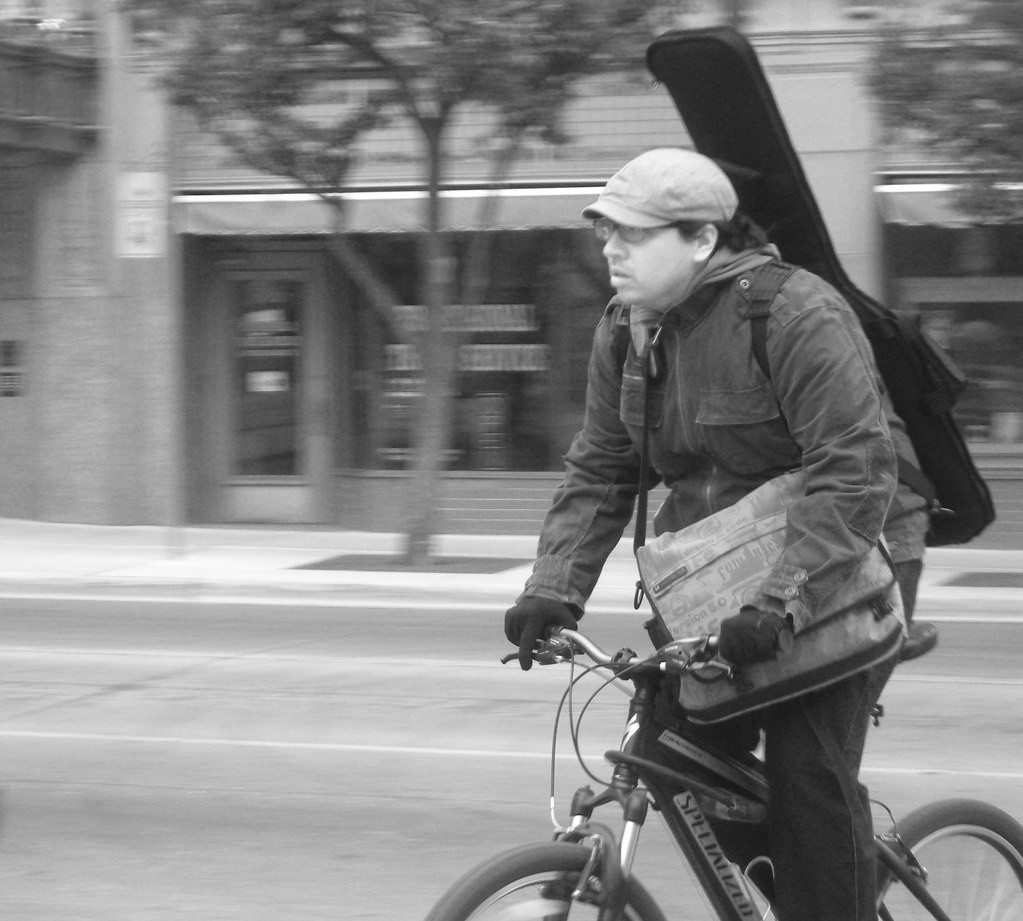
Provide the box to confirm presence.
[502,148,932,921]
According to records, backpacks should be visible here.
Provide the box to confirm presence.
[610,260,999,547]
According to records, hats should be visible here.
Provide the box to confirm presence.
[579,145,740,229]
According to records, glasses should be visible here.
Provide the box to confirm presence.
[594,216,688,245]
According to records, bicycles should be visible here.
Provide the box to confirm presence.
[420,615,1023,921]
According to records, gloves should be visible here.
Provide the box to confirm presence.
[718,605,789,665]
[509,597,579,670]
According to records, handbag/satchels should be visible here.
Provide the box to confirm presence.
[632,467,909,729]
